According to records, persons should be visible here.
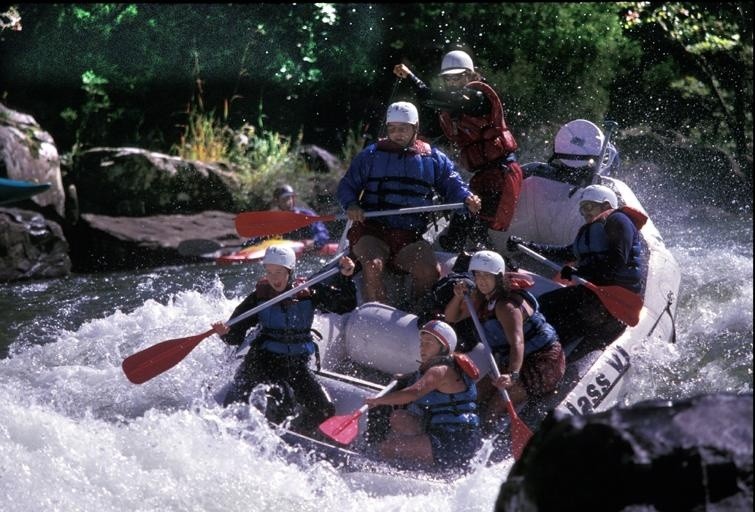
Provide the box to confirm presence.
[392,51,522,229]
[362,319,481,461]
[337,100,491,307]
[242,185,330,249]
[447,251,566,413]
[506,184,651,359]
[210,246,357,422]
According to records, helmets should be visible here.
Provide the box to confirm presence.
[578,183,620,210]
[273,184,296,201]
[385,101,420,128]
[418,319,457,354]
[468,250,505,277]
[437,50,475,77]
[263,244,297,271]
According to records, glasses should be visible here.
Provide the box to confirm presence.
[385,124,411,133]
[578,201,600,216]
[441,73,467,83]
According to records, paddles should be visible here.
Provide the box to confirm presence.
[465,291,533,465]
[319,377,400,445]
[512,239,643,326]
[122,269,343,384]
[234,201,473,238]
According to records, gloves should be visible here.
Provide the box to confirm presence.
[507,234,529,252]
[561,264,576,281]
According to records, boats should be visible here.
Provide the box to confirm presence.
[202,174,683,500]
[213,238,341,265]
